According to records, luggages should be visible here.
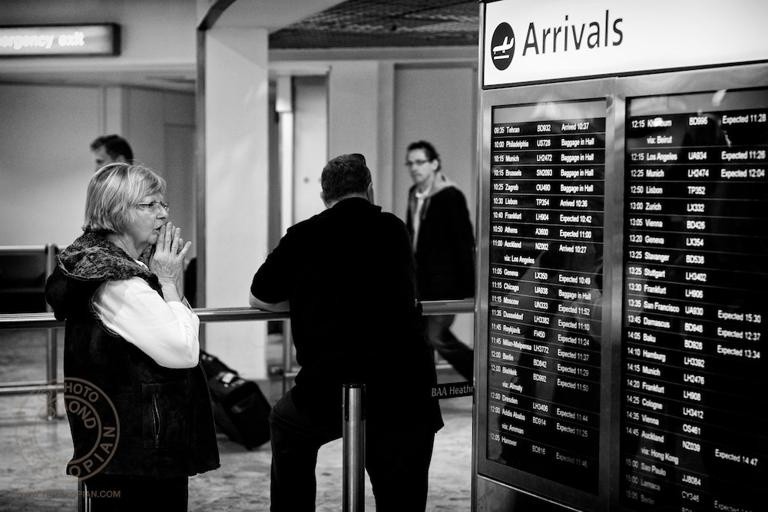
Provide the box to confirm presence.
[208,370,272,450]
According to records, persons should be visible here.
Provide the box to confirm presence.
[404,138,476,388]
[244,151,445,512]
[87,131,135,173]
[43,161,221,512]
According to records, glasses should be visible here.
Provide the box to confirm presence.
[405,159,429,167]
[138,200,169,212]
